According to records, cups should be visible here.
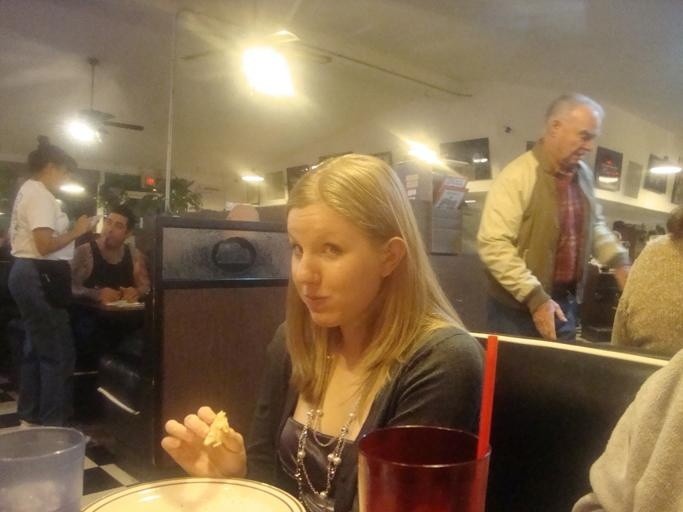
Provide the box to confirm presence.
[357,426,493,510]
[0,425,88,511]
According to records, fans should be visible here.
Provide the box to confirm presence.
[67,56,144,135]
[180,1,331,67]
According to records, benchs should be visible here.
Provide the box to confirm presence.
[466,329,672,512]
[91,345,149,459]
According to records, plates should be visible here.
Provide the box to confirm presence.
[85,477,304,512]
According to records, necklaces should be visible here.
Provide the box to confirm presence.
[293,341,367,511]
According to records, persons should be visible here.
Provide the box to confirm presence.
[159,151,492,511]
[474,91,635,342]
[225,204,259,222]
[68,204,151,309]
[609,200,682,361]
[569,346,682,512]
[5,134,101,428]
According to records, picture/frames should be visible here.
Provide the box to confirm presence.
[438,138,492,182]
[593,145,683,205]
[228,165,309,205]
